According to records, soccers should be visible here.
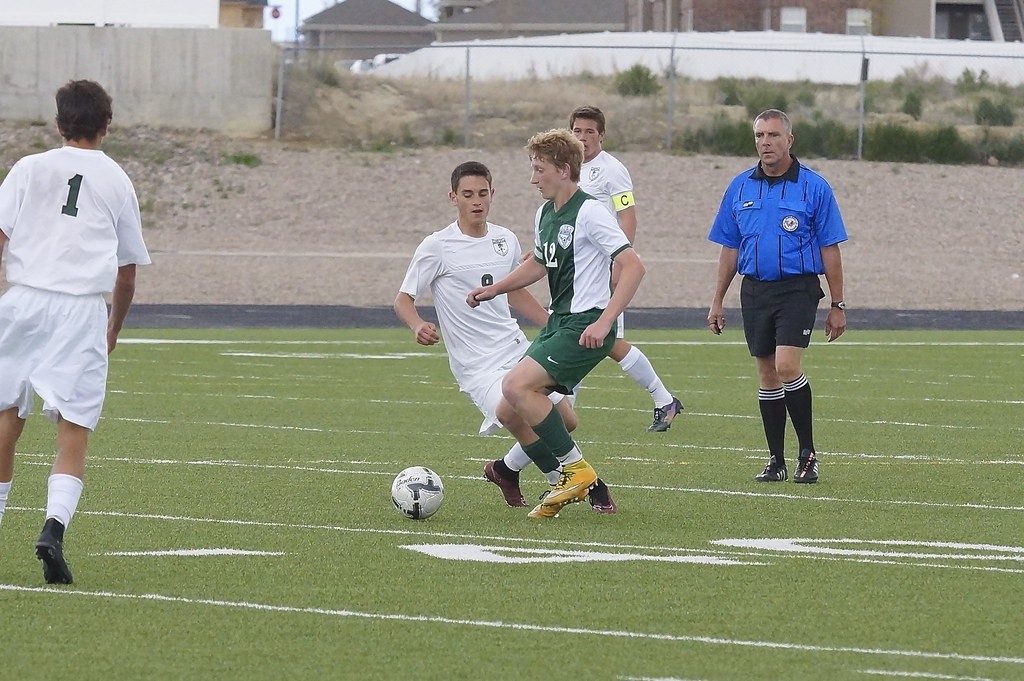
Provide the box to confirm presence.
[391,465,447,522]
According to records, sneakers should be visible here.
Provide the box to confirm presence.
[35,519,73,584]
[648,397,684,432]
[589,479,617,513]
[756,455,788,482]
[483,458,529,507]
[794,449,818,483]
[527,459,597,518]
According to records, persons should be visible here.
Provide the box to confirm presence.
[554,104,685,431]
[0,79,152,586]
[465,127,646,520]
[707,107,847,484]
[394,160,618,515]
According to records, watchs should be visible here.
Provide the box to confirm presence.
[830,300,846,311]
